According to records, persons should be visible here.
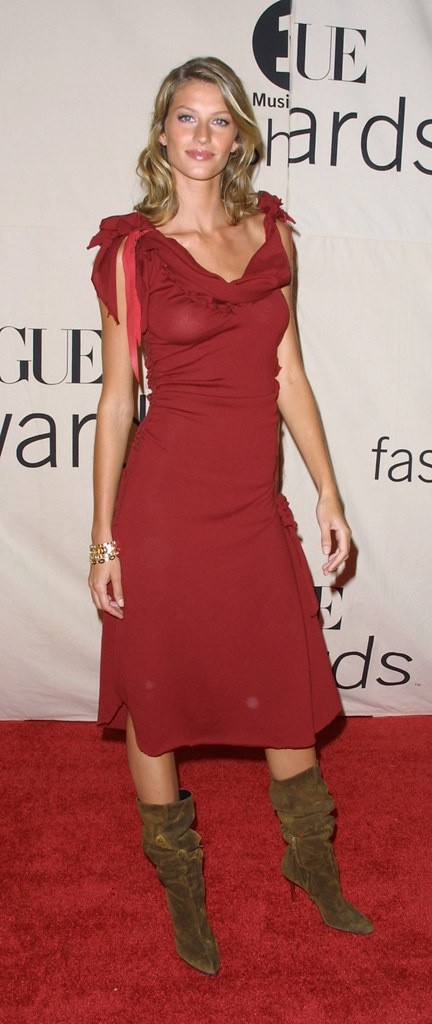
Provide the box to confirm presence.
[87,57,372,975]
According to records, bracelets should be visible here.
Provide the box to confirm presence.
[90,540,119,564]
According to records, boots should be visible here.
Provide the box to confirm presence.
[268,759,375,935]
[136,788,220,975]
